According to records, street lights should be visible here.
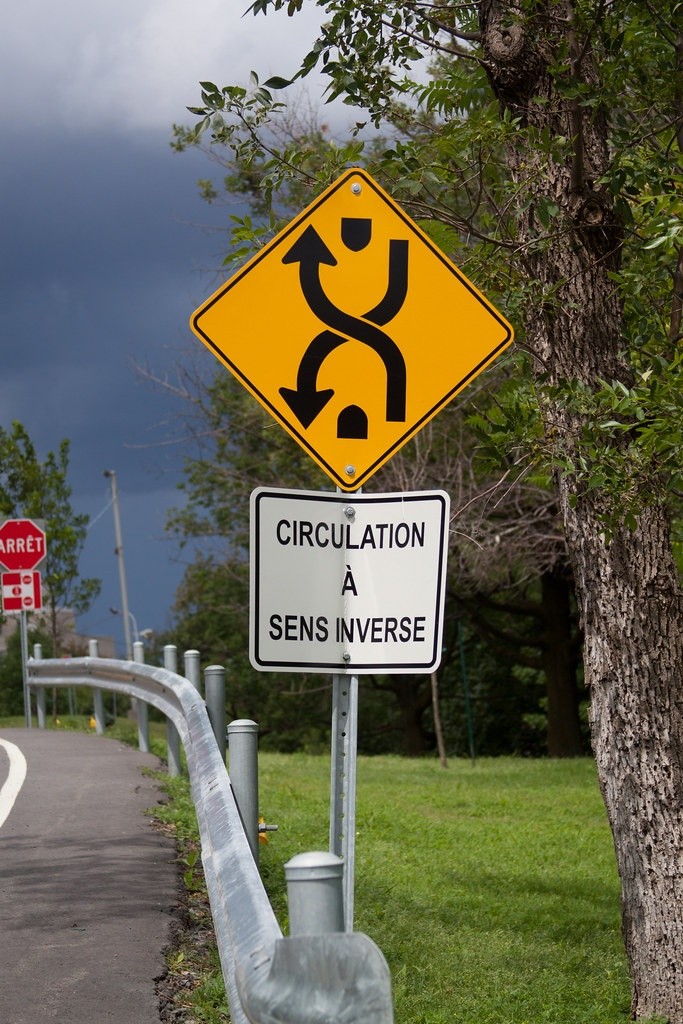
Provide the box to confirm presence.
[108,604,139,641]
[103,468,135,662]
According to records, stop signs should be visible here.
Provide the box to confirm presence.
[1,517,47,573]
